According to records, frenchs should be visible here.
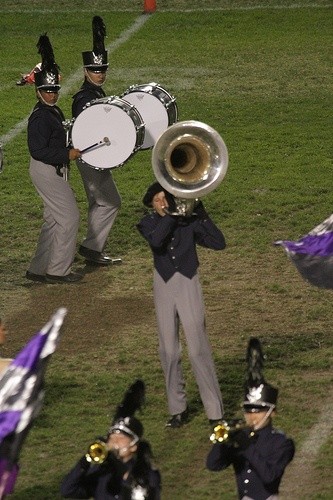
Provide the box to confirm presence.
[151,120,229,217]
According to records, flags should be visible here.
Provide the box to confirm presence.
[0,306,68,500]
[273,212,333,284]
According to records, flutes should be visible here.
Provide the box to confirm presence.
[208,419,258,444]
[85,439,119,464]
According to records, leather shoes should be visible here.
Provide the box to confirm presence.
[25,271,82,283]
[165,406,187,428]
[78,245,121,266]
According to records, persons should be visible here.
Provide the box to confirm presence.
[60,417,162,500]
[25,69,83,284]
[206,380,295,500]
[71,51,122,266]
[136,181,226,428]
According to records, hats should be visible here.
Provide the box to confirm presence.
[141,181,163,208]
[33,65,60,90]
[239,380,279,413]
[105,414,143,439]
[81,50,109,73]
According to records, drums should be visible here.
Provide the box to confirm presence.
[69,95,146,171]
[120,82,179,151]
